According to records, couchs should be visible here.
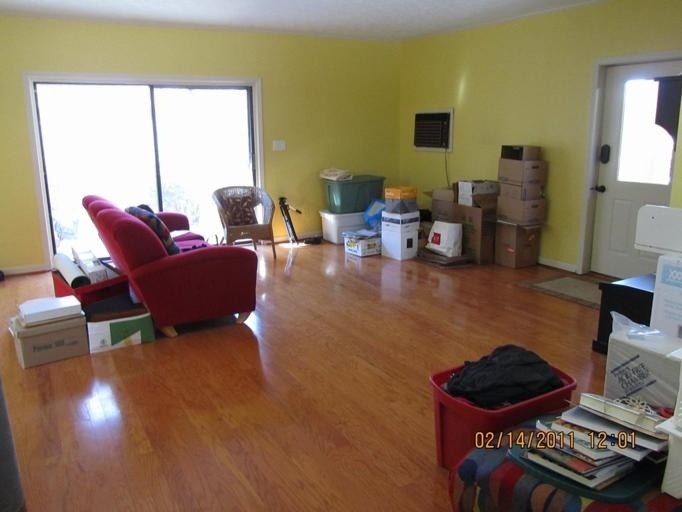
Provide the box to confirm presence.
[81,194,258,338]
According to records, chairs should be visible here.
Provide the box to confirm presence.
[212,185,277,260]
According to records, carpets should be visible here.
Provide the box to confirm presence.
[519,272,601,311]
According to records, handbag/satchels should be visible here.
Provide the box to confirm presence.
[425,218,465,258]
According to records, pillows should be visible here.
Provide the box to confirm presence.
[138,204,154,215]
[220,195,258,226]
[125,206,181,255]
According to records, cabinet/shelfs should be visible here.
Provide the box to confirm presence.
[592,272,656,357]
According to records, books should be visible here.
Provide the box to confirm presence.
[524,392,669,492]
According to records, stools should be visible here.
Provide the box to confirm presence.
[446,399,682,512]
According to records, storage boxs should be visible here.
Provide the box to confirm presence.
[51,257,130,308]
[429,362,578,477]
[317,145,549,270]
[87,301,156,354]
[11,315,88,370]
